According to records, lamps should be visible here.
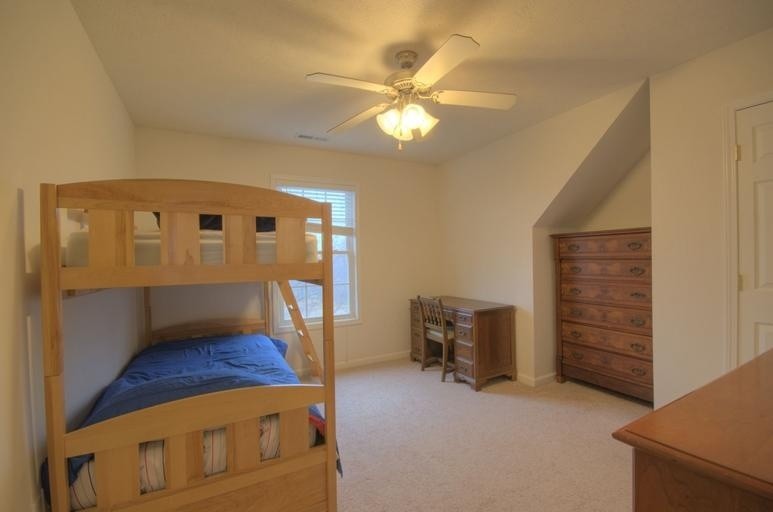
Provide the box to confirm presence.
[375,94,441,150]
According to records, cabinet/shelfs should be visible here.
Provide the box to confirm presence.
[548,226,653,404]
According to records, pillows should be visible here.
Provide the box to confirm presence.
[68,411,317,509]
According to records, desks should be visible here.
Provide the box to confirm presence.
[612,347,772,512]
[408,294,517,392]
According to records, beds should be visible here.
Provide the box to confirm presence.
[39,178,342,512]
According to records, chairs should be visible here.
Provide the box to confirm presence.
[416,295,454,382]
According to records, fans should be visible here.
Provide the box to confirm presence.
[305,32,518,135]
